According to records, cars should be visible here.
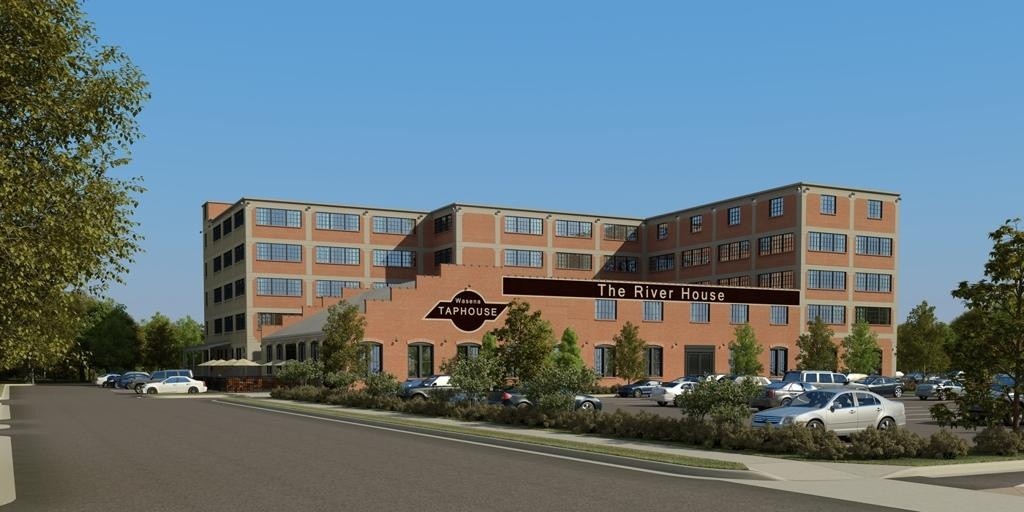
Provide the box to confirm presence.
[751,389,906,436]
[95,370,207,394]
[397,375,601,411]
[990,374,1024,426]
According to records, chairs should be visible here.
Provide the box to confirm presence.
[221,375,264,393]
